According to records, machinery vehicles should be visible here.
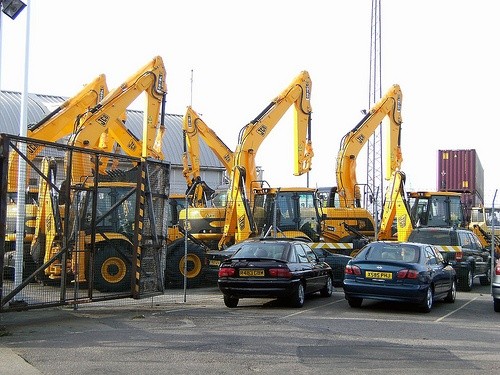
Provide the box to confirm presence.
[3,57,500,294]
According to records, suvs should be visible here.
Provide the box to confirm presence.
[407,225,492,292]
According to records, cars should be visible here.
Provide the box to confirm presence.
[217,241,334,308]
[491,257,500,314]
[204,238,353,287]
[342,240,457,313]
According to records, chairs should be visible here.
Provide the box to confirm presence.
[254,248,268,257]
